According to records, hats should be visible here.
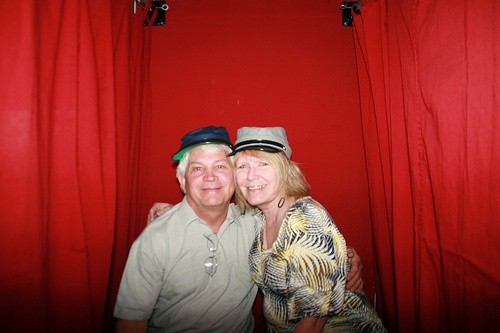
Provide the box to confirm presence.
[226,127,292,162]
[172,127,233,162]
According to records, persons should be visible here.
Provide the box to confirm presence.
[147,126,387,333]
[113,125,364,333]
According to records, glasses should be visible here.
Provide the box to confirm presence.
[202,233,218,277]
